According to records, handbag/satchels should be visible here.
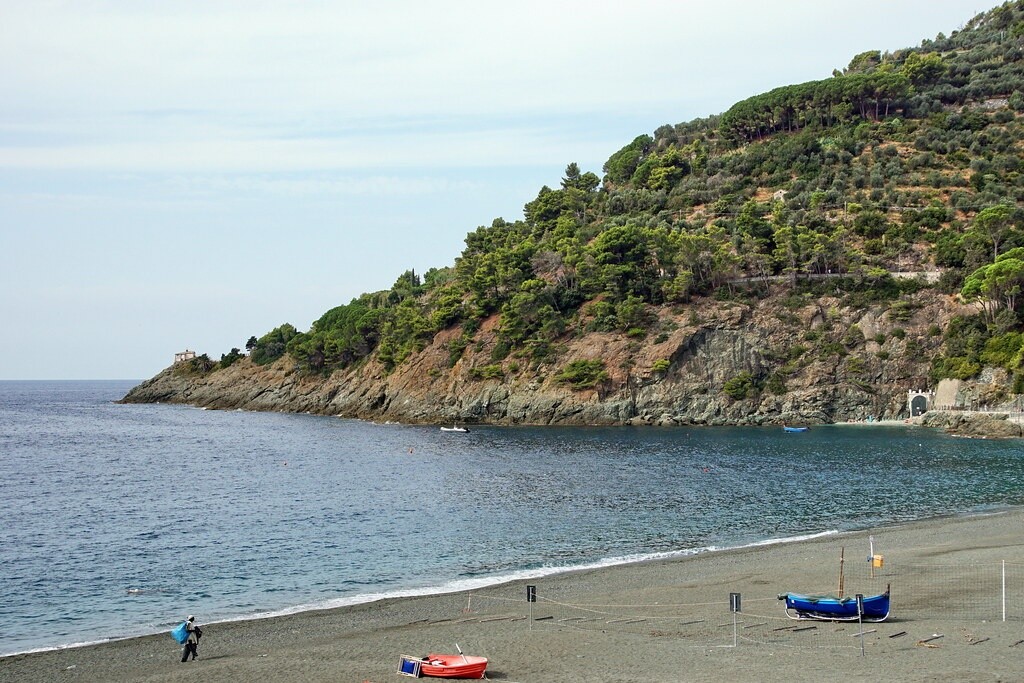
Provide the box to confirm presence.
[171,622,190,645]
[194,627,202,638]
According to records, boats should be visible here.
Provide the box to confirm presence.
[440,421,471,434]
[419,652,489,680]
[776,581,891,622]
[783,426,812,432]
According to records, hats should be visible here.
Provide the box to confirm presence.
[188,615,194,619]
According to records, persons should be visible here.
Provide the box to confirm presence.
[180,615,198,662]
